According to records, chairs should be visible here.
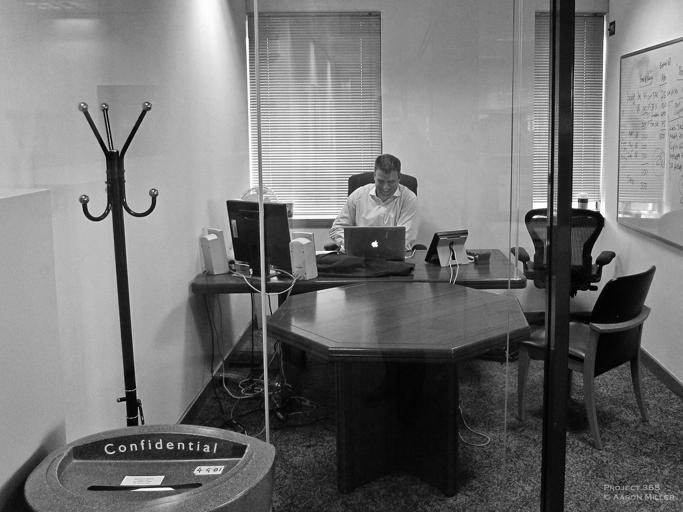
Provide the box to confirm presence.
[347,171,418,197]
[510,205,657,451]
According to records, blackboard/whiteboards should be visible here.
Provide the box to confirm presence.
[617,38,683,250]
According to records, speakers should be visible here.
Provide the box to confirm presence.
[289,232,318,280]
[201,228,229,275]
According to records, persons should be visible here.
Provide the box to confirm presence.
[328,154,417,253]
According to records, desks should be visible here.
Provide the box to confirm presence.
[193,247,527,497]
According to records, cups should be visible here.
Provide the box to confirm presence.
[577,197,588,212]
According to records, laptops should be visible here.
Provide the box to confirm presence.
[343,224,406,261]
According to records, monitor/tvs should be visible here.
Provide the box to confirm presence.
[225,197,292,281]
